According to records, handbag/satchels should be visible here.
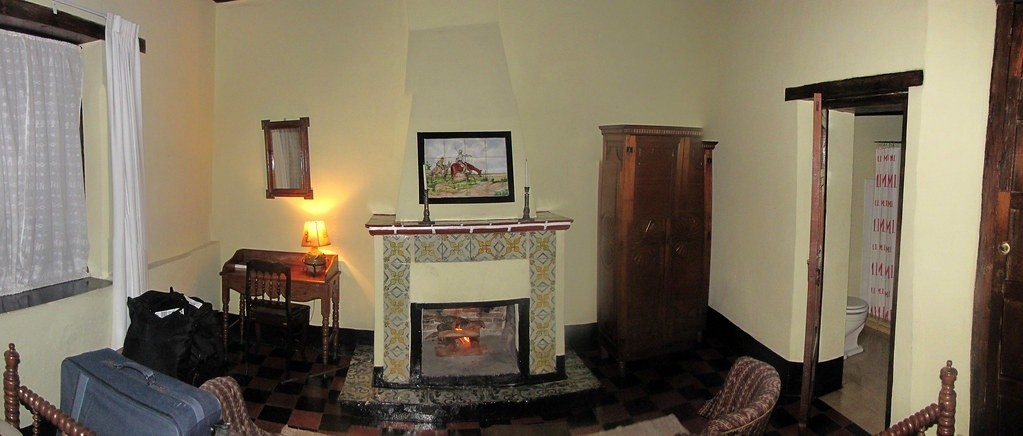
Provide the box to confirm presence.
[123,287,225,387]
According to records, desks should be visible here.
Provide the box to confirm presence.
[219,248,342,381]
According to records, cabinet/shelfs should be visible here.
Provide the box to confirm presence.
[599,125,717,366]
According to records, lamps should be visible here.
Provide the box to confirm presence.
[300,220,332,275]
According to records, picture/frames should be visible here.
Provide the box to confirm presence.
[417,130,518,206]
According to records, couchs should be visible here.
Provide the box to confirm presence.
[588,355,783,436]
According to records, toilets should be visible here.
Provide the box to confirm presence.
[843,294,870,360]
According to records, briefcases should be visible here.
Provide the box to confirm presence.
[55,348,231,436]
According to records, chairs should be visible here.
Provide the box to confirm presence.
[245,259,311,370]
